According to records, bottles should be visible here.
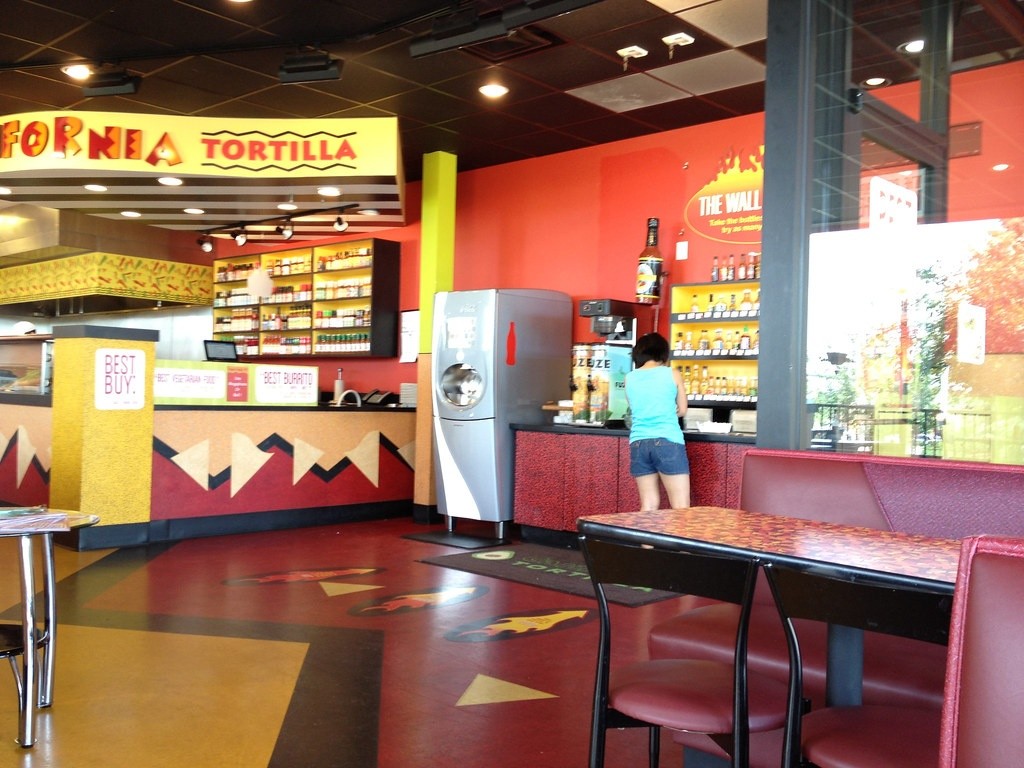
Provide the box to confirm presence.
[691,295,698,312]
[754,252,761,279]
[713,329,724,350]
[698,330,709,350]
[678,364,757,397]
[707,294,727,311]
[729,294,737,311]
[214,248,373,355]
[719,254,735,281]
[737,254,747,280]
[675,332,694,350]
[747,252,755,280]
[636,217,664,305]
[739,289,760,310]
[711,256,719,282]
[753,329,759,350]
[726,328,751,350]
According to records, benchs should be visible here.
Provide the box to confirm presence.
[641,449,1023,711]
[937,526,1023,767]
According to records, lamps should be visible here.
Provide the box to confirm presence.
[409,0,600,58]
[278,45,345,83]
[196,209,348,252]
[82,62,138,98]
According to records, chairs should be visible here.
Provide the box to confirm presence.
[761,561,957,767]
[578,534,815,768]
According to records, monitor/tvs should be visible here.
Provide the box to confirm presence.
[204,340,238,362]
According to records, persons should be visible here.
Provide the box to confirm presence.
[625,332,691,553]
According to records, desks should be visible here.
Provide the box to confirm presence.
[576,505,1023,768]
[0,506,101,748]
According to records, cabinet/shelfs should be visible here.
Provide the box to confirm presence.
[210,238,397,358]
[665,275,762,410]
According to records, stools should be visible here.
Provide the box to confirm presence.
[0,623,49,745]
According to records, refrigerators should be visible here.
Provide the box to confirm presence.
[430,288,573,539]
